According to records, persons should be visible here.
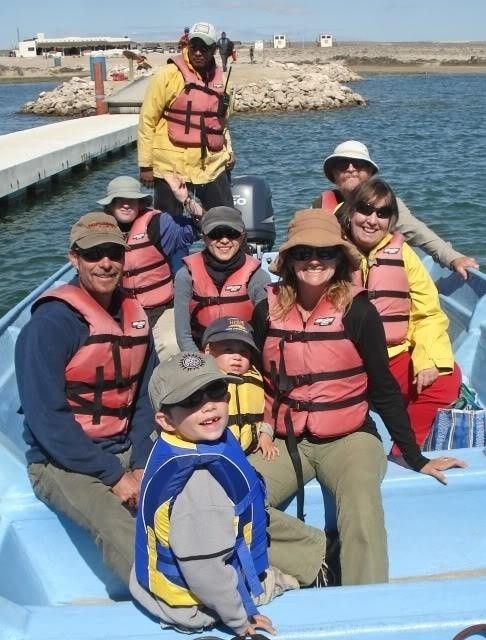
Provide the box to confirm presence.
[217,32,234,72]
[14,210,161,588]
[179,27,190,50]
[128,351,299,637]
[96,164,206,363]
[137,22,236,226]
[244,207,468,587]
[202,315,280,461]
[268,140,480,280]
[174,206,271,351]
[333,178,462,456]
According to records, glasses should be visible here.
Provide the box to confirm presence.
[291,246,338,262]
[209,230,239,242]
[176,383,226,408]
[358,203,390,217]
[77,246,122,260]
[337,160,364,169]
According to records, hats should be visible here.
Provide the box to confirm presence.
[323,141,379,181]
[200,206,245,234]
[97,175,153,209]
[268,210,362,277]
[188,23,217,47]
[69,212,131,251]
[202,316,261,354]
[147,353,242,411]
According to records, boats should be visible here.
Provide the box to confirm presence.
[0,175,483,640]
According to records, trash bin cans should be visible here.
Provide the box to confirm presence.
[53,56,61,66]
[90,55,106,81]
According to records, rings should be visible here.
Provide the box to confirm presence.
[444,458,448,462]
